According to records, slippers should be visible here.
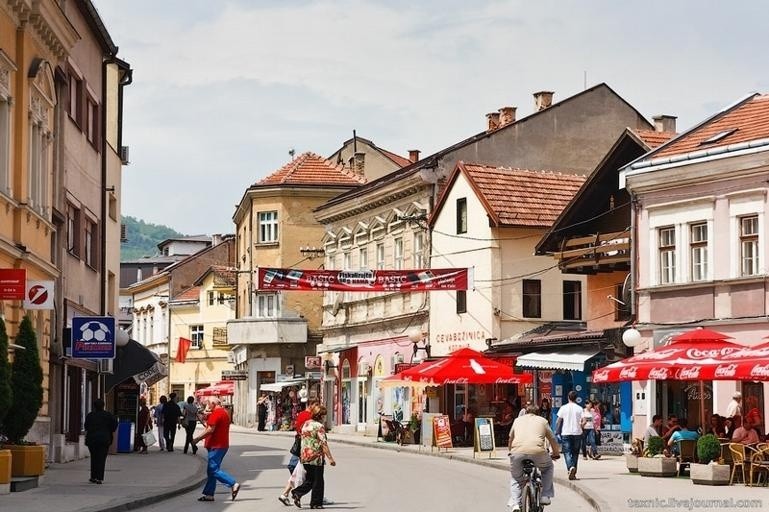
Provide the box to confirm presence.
[197,497,215,502]
[279,498,293,507]
[232,485,241,501]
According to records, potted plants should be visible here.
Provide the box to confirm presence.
[1,315,14,484]
[638,434,676,479]
[689,433,730,486]
[9,309,44,477]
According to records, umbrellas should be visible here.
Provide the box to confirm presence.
[687,326,769,383]
[592,323,745,436]
[394,345,534,442]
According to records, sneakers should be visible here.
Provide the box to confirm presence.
[567,467,576,480]
[592,455,600,460]
[539,497,552,506]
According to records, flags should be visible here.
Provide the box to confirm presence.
[175,336,191,364]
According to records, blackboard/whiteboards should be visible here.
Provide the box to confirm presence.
[474,417,496,454]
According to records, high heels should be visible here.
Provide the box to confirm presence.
[586,450,593,458]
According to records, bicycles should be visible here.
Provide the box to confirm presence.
[505,455,561,512]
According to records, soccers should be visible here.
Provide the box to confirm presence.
[78,320,111,343]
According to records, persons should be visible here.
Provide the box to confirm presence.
[506,404,560,511]
[279,403,318,506]
[703,391,759,465]
[191,396,246,500]
[300,385,307,410]
[292,406,335,511]
[84,398,118,482]
[645,412,700,460]
[255,400,267,431]
[134,391,200,454]
[519,390,603,478]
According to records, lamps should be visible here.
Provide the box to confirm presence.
[409,324,430,357]
[620,316,641,349]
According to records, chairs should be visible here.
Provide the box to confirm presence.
[673,437,769,487]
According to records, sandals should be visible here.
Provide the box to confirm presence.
[292,495,302,509]
[310,505,324,510]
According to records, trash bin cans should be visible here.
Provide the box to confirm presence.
[117,421,132,454]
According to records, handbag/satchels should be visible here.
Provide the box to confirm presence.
[290,434,301,457]
[141,423,157,448]
